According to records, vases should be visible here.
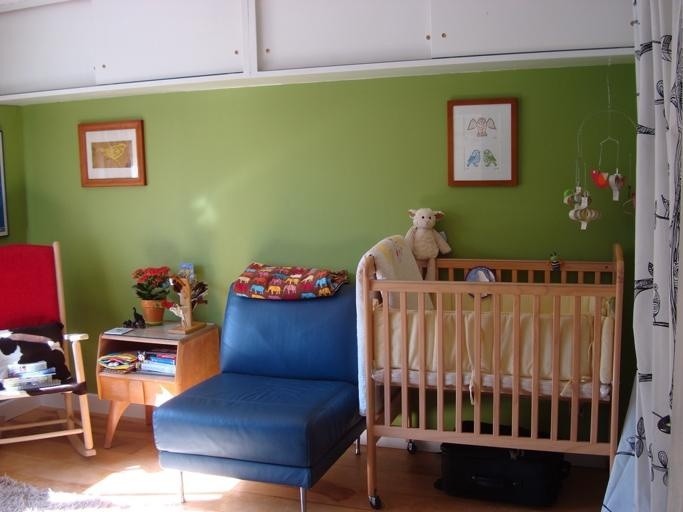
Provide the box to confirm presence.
[139,299,165,325]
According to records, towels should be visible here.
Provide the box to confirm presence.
[233,263,348,299]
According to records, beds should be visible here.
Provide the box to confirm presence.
[361,243,626,507]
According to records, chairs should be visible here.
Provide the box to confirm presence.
[151,285,400,512]
[0,239,97,457]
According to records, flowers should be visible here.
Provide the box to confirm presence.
[128,266,175,309]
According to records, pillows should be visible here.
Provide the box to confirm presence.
[0,322,73,390]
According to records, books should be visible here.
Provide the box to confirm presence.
[104,327,133,335]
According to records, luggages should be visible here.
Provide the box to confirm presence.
[433,420,571,509]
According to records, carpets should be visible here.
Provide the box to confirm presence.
[0,474,138,512]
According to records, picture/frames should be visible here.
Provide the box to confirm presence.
[447,97,518,188]
[0,130,9,236]
[78,120,146,187]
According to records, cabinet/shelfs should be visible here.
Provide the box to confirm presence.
[95,324,220,448]
